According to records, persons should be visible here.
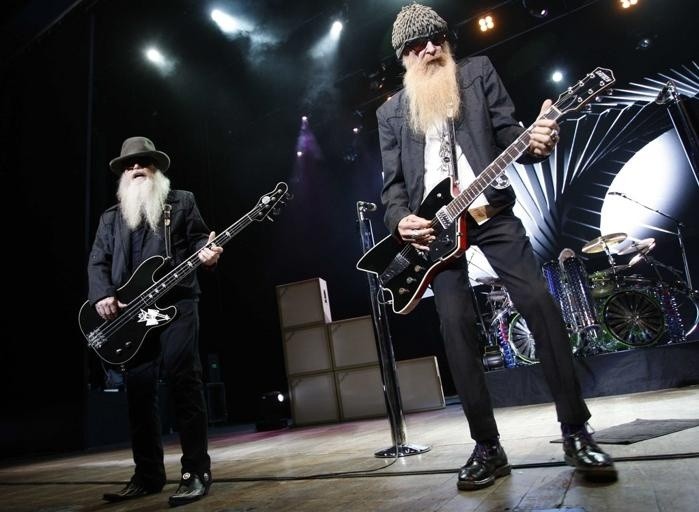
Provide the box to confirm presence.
[371,2,618,491]
[82,134,227,507]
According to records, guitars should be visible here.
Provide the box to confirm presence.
[78,182,294,365]
[355,66,616,316]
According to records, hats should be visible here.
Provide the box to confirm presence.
[392,2,448,58]
[109,137,171,174]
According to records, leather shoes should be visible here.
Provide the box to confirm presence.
[563,435,617,476]
[457,441,510,491]
[169,474,212,503]
[104,478,163,499]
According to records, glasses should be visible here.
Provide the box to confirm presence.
[404,30,445,53]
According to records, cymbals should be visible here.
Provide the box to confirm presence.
[622,273,651,283]
[628,243,656,267]
[617,236,656,256]
[582,233,627,253]
[587,265,628,281]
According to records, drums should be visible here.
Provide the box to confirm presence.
[660,287,699,342]
[590,290,666,351]
[508,311,579,364]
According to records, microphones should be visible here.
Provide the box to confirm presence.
[357,200,377,211]
[609,192,621,194]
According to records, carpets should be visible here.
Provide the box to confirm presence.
[549,418,699,444]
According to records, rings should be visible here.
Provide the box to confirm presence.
[410,228,423,241]
[547,128,559,142]
